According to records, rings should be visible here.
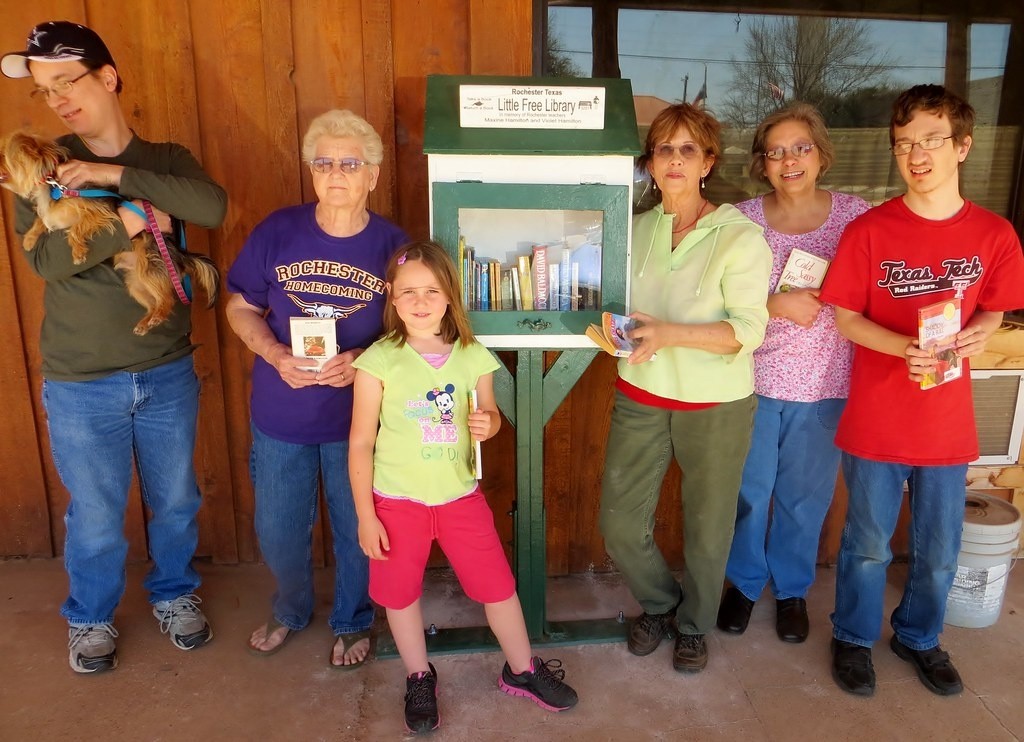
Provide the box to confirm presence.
[341,374,345,380]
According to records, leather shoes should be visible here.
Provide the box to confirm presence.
[892,635,962,696]
[832,637,875,696]
[775,595,810,644]
[719,588,755,633]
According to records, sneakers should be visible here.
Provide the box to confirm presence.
[404,662,440,732]
[627,600,677,657]
[497,656,579,711]
[672,631,709,671]
[153,593,213,652]
[67,621,120,673]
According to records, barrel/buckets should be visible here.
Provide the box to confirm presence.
[942,491,1022,629]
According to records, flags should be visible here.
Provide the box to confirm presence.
[771,85,784,101]
[692,84,707,108]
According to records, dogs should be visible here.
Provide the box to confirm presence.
[0,129,222,337]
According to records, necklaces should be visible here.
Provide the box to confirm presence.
[672,199,709,234]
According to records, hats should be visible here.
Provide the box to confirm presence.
[1,19,116,80]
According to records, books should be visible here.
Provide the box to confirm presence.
[289,317,337,373]
[774,248,830,293]
[918,298,963,390]
[585,312,658,361]
[458,235,597,310]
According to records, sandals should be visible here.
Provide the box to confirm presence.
[329,628,370,669]
[247,611,313,655]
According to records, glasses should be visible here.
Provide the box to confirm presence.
[31,69,91,102]
[888,133,954,156]
[650,144,706,159]
[309,156,372,173]
[758,143,817,160]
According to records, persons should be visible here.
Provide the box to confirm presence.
[225,108,419,670]
[0,20,229,675]
[819,83,1024,694]
[599,99,773,675]
[616,328,638,344]
[348,232,579,732]
[718,100,873,642]
